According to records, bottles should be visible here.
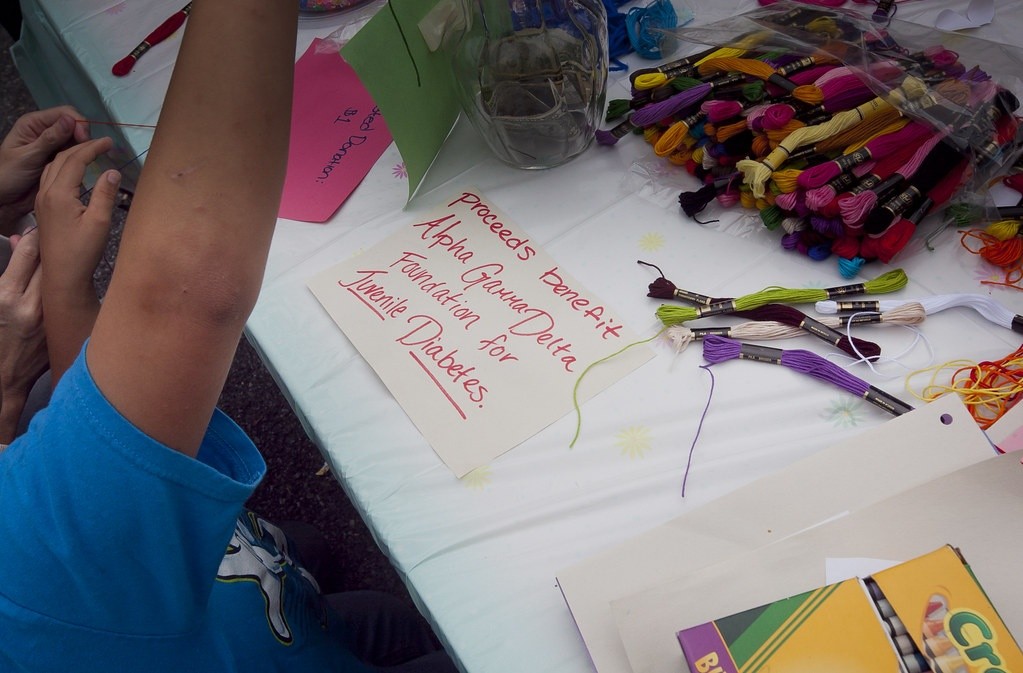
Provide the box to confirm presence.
[443,0,608,168]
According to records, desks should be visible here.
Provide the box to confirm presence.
[10,0,1023,673]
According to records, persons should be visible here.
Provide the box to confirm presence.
[0,0,455,673]
